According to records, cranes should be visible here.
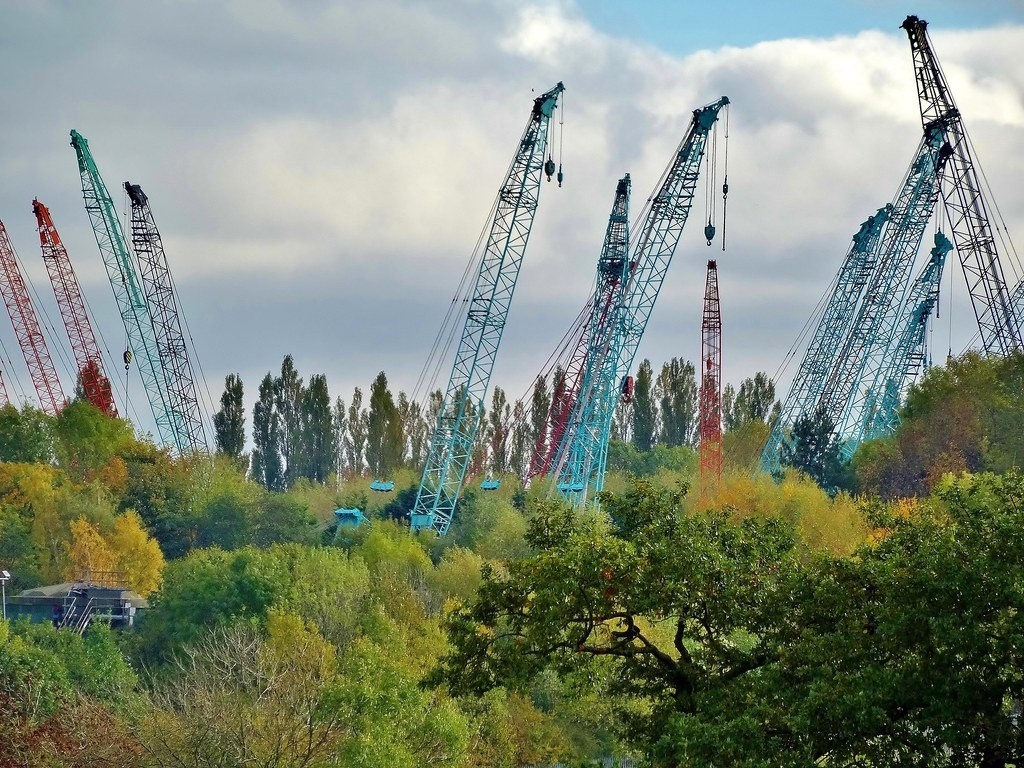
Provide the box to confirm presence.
[746,15,1024,511]
[396,80,734,542]
[1,124,215,476]
